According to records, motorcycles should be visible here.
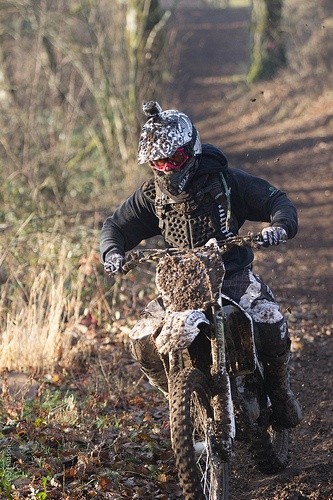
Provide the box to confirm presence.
[110,233,291,500]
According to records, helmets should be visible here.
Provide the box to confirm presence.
[136,100,201,165]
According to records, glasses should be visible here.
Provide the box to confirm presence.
[148,123,197,172]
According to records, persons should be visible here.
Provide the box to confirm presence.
[98,109,305,427]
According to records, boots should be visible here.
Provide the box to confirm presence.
[140,366,168,400]
[255,336,305,428]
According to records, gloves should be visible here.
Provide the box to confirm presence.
[262,226,287,246]
[103,252,123,277]
